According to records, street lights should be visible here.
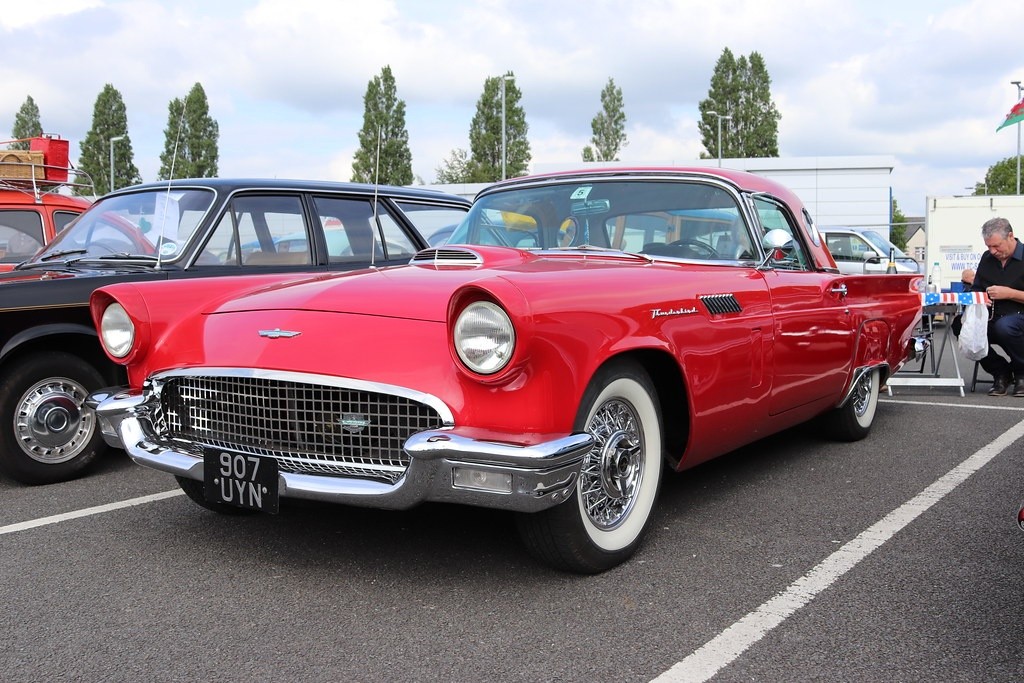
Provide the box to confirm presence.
[502,76,515,182]
[706,111,732,168]
[110,137,124,192]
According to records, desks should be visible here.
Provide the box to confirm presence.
[885,292,992,397]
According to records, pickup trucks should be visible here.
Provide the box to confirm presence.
[90,166,925,575]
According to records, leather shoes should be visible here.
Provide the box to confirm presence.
[988,369,1014,396]
[1013,379,1024,397]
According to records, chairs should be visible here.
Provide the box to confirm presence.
[863,256,937,373]
[833,241,846,253]
[243,252,308,265]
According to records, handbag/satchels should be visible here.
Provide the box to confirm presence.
[957,304,989,360]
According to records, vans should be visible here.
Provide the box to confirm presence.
[815,225,918,276]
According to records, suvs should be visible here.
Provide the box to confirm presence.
[0,186,160,284]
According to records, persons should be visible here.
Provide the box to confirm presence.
[972,218,1024,397]
[950,270,974,341]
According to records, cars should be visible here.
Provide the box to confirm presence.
[233,229,414,261]
[366,182,497,255]
[0,177,515,487]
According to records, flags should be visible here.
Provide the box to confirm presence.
[995,97,1024,131]
